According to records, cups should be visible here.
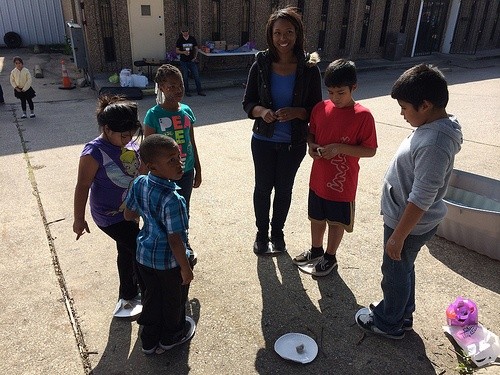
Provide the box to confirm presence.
[186,48,190,55]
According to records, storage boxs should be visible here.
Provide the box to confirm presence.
[199,40,239,53]
[143,57,161,63]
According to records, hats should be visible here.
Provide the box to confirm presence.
[182,26,188,31]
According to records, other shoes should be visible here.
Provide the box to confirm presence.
[30,114,35,118]
[21,114,27,118]
[142,337,159,355]
[159,316,195,349]
[187,242,194,262]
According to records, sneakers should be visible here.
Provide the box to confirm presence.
[271,230,286,252]
[312,257,337,276]
[357,314,405,339]
[293,250,324,265]
[369,301,413,330]
[253,231,269,254]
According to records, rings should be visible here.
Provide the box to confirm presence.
[285,113,287,116]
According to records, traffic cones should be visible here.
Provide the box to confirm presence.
[58,60,77,90]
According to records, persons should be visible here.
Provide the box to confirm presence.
[73,93,144,301]
[0,83,5,105]
[10,56,36,118]
[124,133,194,353]
[356,60,463,339]
[292,58,378,277]
[242,4,322,254]
[143,65,202,219]
[176,25,207,97]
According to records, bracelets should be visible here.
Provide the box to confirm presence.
[194,57,196,59]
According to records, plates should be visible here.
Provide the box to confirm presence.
[274,333,318,364]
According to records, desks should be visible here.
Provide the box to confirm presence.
[195,46,259,73]
[134,60,181,83]
